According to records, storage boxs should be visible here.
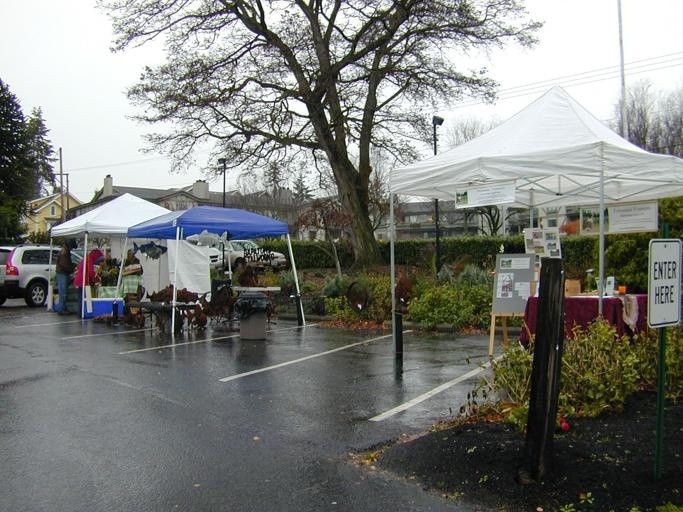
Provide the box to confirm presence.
[54,293,79,312]
[83,298,123,319]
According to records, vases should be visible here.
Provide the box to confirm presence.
[101,277,109,286]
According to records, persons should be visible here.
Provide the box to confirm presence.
[74,249,102,317]
[56,239,77,314]
[124,248,142,275]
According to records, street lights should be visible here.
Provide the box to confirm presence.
[217,159,226,208]
[431,115,444,278]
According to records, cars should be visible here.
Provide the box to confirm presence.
[184,233,225,268]
[222,240,285,273]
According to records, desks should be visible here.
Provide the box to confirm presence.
[522,293,648,348]
[94,285,122,298]
[124,301,196,337]
[223,283,283,326]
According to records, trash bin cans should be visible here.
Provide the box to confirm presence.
[236,292,269,340]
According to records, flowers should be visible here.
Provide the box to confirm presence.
[95,255,117,277]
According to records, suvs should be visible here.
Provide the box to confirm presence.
[1,243,103,307]
